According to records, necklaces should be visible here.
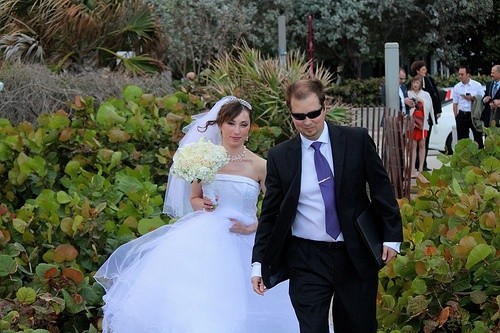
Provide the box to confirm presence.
[227,145,247,161]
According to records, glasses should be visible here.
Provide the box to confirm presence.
[291,106,323,120]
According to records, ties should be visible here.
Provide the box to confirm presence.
[492,83,498,98]
[310,141,342,241]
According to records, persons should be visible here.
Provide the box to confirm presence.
[381,67,415,179]
[452,66,485,149]
[94,96,333,333]
[482,65,500,139]
[407,75,432,178]
[250,79,404,333]
[405,60,442,172]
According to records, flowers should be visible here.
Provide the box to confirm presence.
[172,136,230,212]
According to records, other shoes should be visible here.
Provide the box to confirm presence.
[417,166,432,172]
